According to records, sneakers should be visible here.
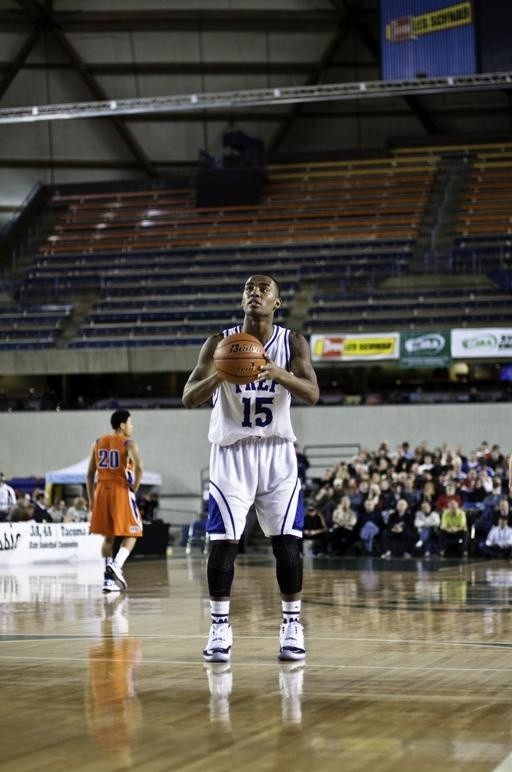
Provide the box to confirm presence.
[105,560,130,589]
[203,625,233,662]
[278,623,307,661]
[102,582,120,591]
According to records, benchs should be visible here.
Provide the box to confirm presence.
[0,135,511,353]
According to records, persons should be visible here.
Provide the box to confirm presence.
[1,472,160,525]
[313,364,511,410]
[2,384,88,410]
[180,272,319,661]
[86,407,144,593]
[299,441,512,562]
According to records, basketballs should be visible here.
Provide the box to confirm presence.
[214,334,266,385]
